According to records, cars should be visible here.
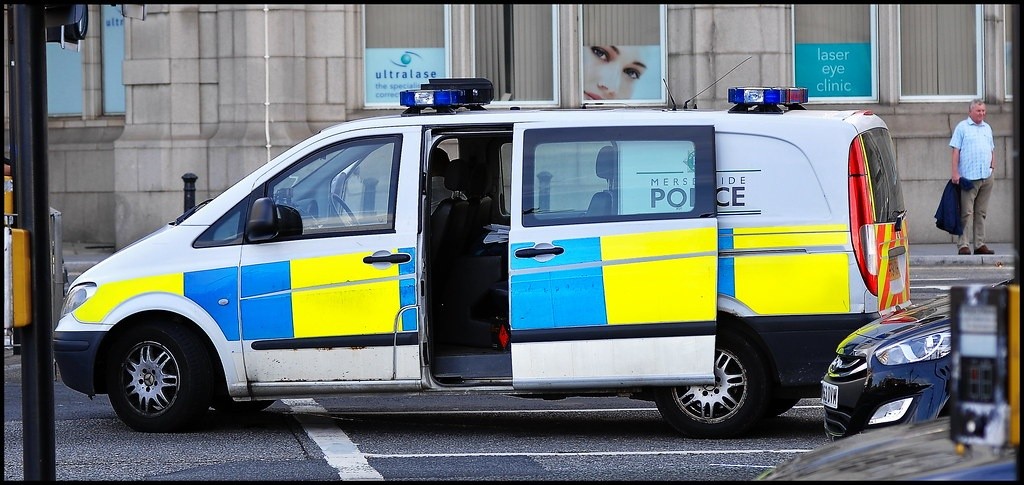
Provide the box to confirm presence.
[746,415,1019,483]
[820,275,1016,440]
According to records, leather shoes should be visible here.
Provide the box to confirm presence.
[958,247,971,254]
[974,245,994,254]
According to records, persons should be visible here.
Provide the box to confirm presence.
[430,147,465,216]
[584,45,646,100]
[950,100,996,255]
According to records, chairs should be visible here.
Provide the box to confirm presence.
[425,158,493,336]
[581,146,618,215]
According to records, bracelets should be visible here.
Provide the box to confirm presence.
[990,167,994,169]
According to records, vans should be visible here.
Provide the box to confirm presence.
[45,76,914,439]
